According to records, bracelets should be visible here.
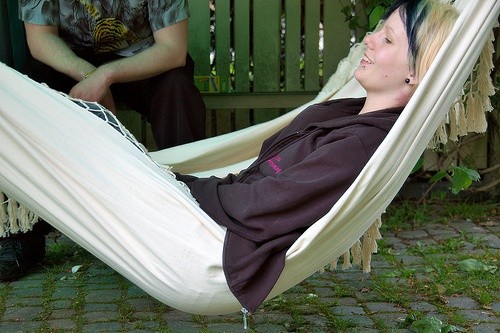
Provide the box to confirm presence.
[82,68,97,80]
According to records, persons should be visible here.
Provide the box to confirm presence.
[0,0,207,280]
[1,0,459,330]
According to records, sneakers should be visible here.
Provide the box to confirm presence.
[0,237,43,282]
